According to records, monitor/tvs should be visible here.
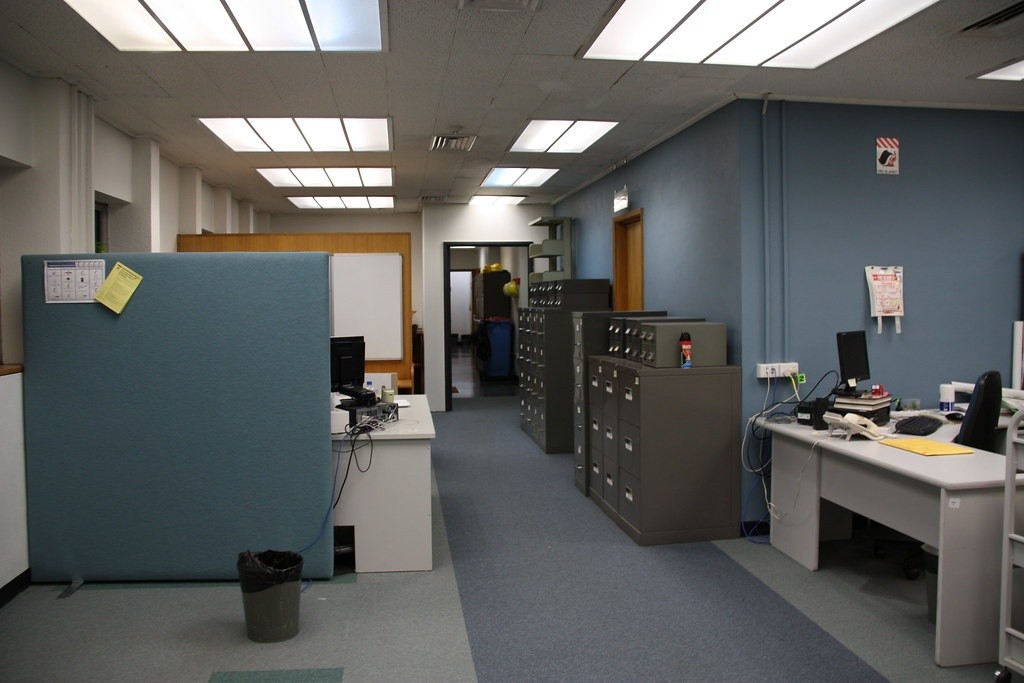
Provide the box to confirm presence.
[833,330,871,397]
[329,335,378,406]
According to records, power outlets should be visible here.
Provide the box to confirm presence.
[758,362,799,378]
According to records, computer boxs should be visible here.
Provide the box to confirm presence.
[827,406,891,426]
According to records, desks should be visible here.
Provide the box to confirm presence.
[747,408,1024,668]
[329,394,438,571]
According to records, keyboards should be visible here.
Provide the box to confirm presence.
[895,416,943,436]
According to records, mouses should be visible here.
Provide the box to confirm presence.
[945,413,964,421]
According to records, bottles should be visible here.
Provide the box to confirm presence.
[679,331,692,369]
[366,381,376,394]
[939,383,954,414]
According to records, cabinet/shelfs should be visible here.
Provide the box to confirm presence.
[516,278,742,545]
[475,270,509,330]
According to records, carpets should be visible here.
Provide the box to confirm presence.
[429,397,890,683]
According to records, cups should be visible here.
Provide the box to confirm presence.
[383,389,394,403]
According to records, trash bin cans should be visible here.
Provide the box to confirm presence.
[482,320,514,377]
[240,551,304,643]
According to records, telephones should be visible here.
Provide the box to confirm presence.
[823,412,884,441]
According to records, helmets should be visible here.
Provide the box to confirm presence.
[503,281,519,298]
[490,263,503,271]
